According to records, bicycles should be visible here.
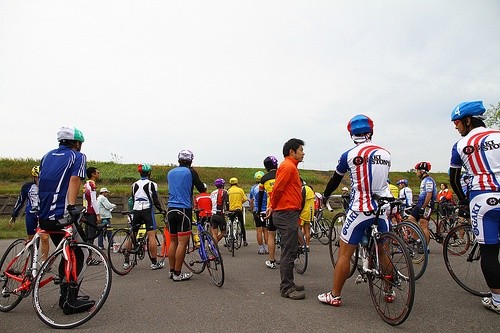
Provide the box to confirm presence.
[184,208,227,288]
[108,209,166,276]
[443,199,500,297]
[302,205,337,245]
[228,198,253,256]
[429,209,470,255]
[389,199,429,282]
[275,219,308,275]
[315,192,416,325]
[0,206,113,329]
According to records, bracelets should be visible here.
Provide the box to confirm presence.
[322,192,329,198]
[421,208,425,211]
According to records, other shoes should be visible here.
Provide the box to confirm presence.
[243,242,248,247]
[223,244,230,247]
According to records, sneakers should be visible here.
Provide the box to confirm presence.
[98,246,106,250]
[280,286,305,300]
[294,284,304,291]
[173,272,193,282]
[481,297,500,314]
[265,249,269,254]
[384,290,396,303]
[63,300,95,315]
[417,241,423,252]
[169,272,173,279]
[123,263,129,269]
[110,247,113,250]
[151,262,165,270]
[451,239,465,247]
[258,249,265,254]
[265,260,276,269]
[59,295,90,309]
[417,248,430,254]
[86,259,102,266]
[317,292,342,307]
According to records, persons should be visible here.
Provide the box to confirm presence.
[264,138,305,300]
[250,170,269,255]
[341,187,351,215]
[208,178,230,261]
[9,164,53,274]
[122,164,168,270]
[317,115,397,307]
[120,196,134,255]
[256,156,310,269]
[387,173,476,252]
[38,125,96,315]
[82,167,102,266]
[167,150,207,282]
[223,176,248,247]
[407,162,437,254]
[96,188,117,250]
[193,183,212,253]
[448,100,500,314]
[298,177,333,254]
[121,192,138,256]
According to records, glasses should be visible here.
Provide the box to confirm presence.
[454,119,460,125]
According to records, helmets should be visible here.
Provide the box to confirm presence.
[31,165,40,176]
[414,161,431,172]
[451,100,486,121]
[137,163,152,173]
[203,183,207,188]
[264,156,278,169]
[387,180,390,184]
[254,171,265,182]
[396,179,408,186]
[347,114,374,142]
[57,126,84,142]
[213,178,226,186]
[229,177,238,184]
[178,150,193,161]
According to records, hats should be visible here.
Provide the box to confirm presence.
[99,187,110,192]
[341,187,348,192]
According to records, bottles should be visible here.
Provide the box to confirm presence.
[193,233,200,248]
[362,233,368,245]
